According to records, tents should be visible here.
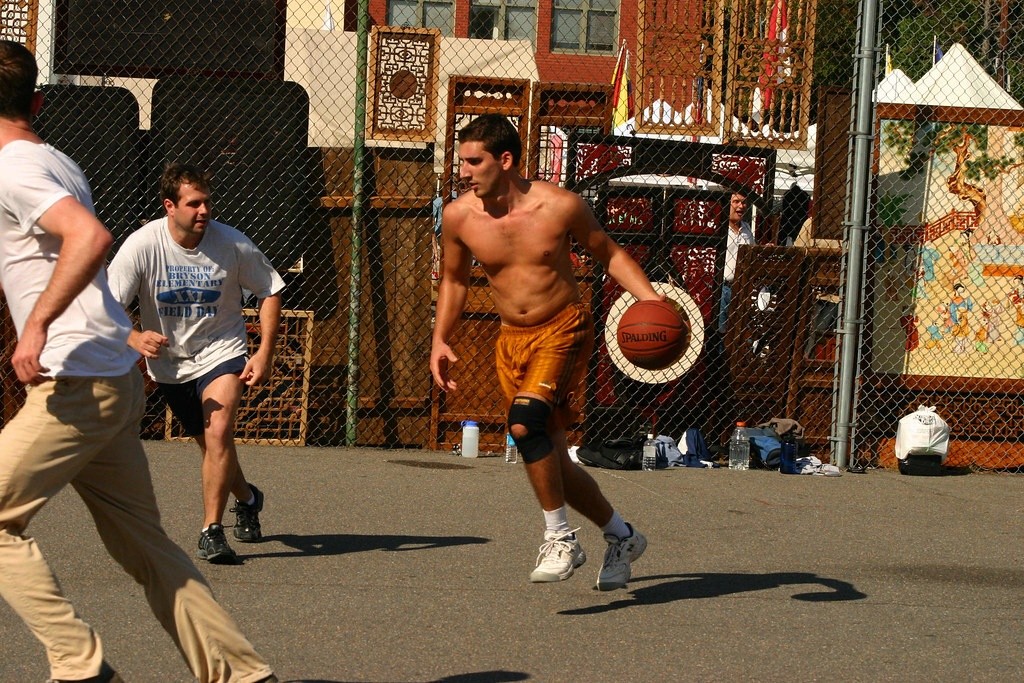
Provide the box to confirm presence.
[611,41,1023,236]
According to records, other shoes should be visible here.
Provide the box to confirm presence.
[45,660,124,683]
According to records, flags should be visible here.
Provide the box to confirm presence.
[687,54,704,182]
[611,40,634,128]
[936,45,942,62]
[751,0,791,124]
[887,54,892,74]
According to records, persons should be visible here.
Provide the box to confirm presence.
[430,114,689,591]
[795,217,844,358]
[106,163,287,560]
[0,38,279,683]
[719,192,775,352]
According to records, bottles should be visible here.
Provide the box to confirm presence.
[729,422,750,469]
[506,431,517,463]
[781,427,795,474]
[460,420,479,458]
[643,434,656,470]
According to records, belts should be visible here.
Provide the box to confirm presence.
[725,280,733,287]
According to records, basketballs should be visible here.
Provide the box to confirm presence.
[615,299,687,371]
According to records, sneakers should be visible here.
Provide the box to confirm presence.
[229,482,264,542]
[197,523,238,563]
[596,522,647,590]
[530,527,587,582]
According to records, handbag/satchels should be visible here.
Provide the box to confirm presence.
[653,435,686,468]
[746,427,783,471]
[898,453,975,476]
[576,436,648,469]
[677,429,720,468]
